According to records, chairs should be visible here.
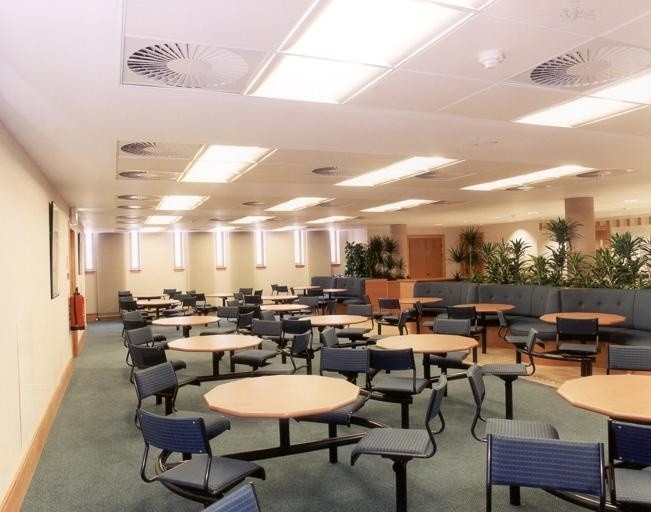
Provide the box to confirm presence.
[128,343,198,414]
[117,278,649,384]
[136,408,265,508]
[482,327,539,419]
[484,432,607,510]
[203,482,261,510]
[369,347,431,430]
[291,347,372,464]
[132,361,232,464]
[350,373,449,510]
[466,365,561,506]
[606,417,650,511]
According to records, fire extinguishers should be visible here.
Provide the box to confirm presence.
[68,286,85,331]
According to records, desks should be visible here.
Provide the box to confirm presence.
[203,374,361,448]
[557,373,650,424]
